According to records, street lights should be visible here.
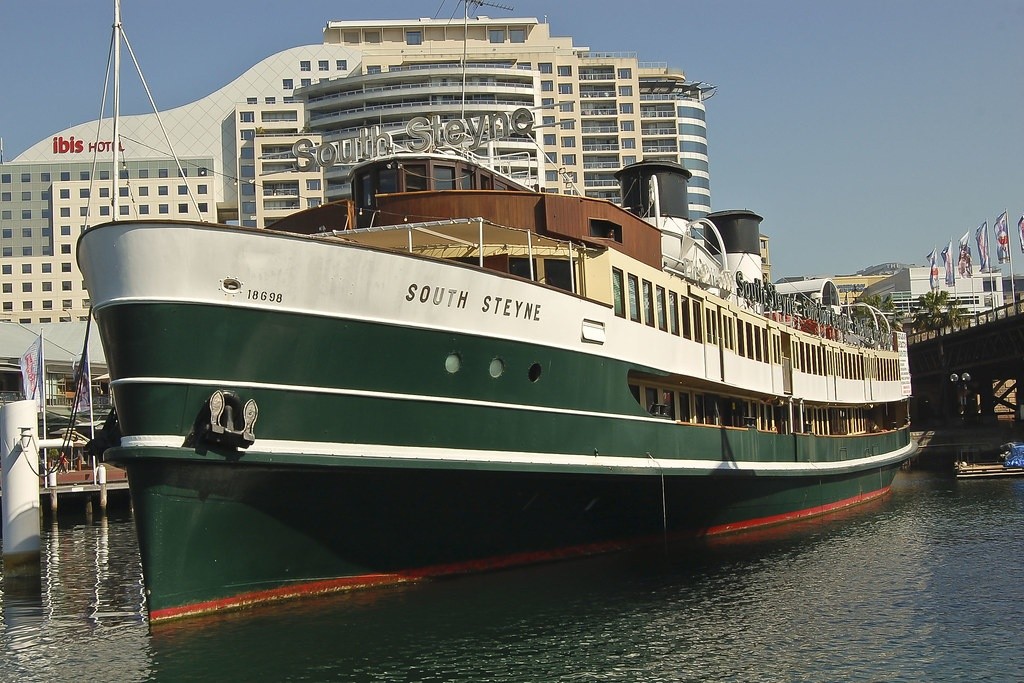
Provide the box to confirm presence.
[950,372,971,423]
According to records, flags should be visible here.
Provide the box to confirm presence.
[927,249,939,289]
[958,232,974,278]
[941,241,956,287]
[976,221,992,273]
[993,212,1011,264]
[1018,215,1024,252]
[20,334,44,413]
[72,355,91,413]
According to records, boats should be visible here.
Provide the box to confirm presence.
[72,0,937,635]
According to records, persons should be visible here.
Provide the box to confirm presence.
[59,452,69,475]
